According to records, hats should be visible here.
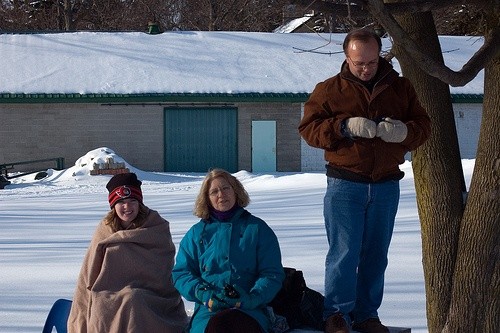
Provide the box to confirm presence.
[106,173,142,210]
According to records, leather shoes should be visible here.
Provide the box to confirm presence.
[325,313,349,333]
[352,319,389,333]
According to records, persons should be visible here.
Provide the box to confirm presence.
[66,172,189,333]
[297,28,433,333]
[170,168,286,333]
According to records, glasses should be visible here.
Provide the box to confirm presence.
[348,56,379,68]
[208,185,233,196]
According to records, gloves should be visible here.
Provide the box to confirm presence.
[347,117,377,138]
[221,283,256,310]
[376,117,407,142]
[197,282,231,312]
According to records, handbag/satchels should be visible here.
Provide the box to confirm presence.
[266,267,325,331]
